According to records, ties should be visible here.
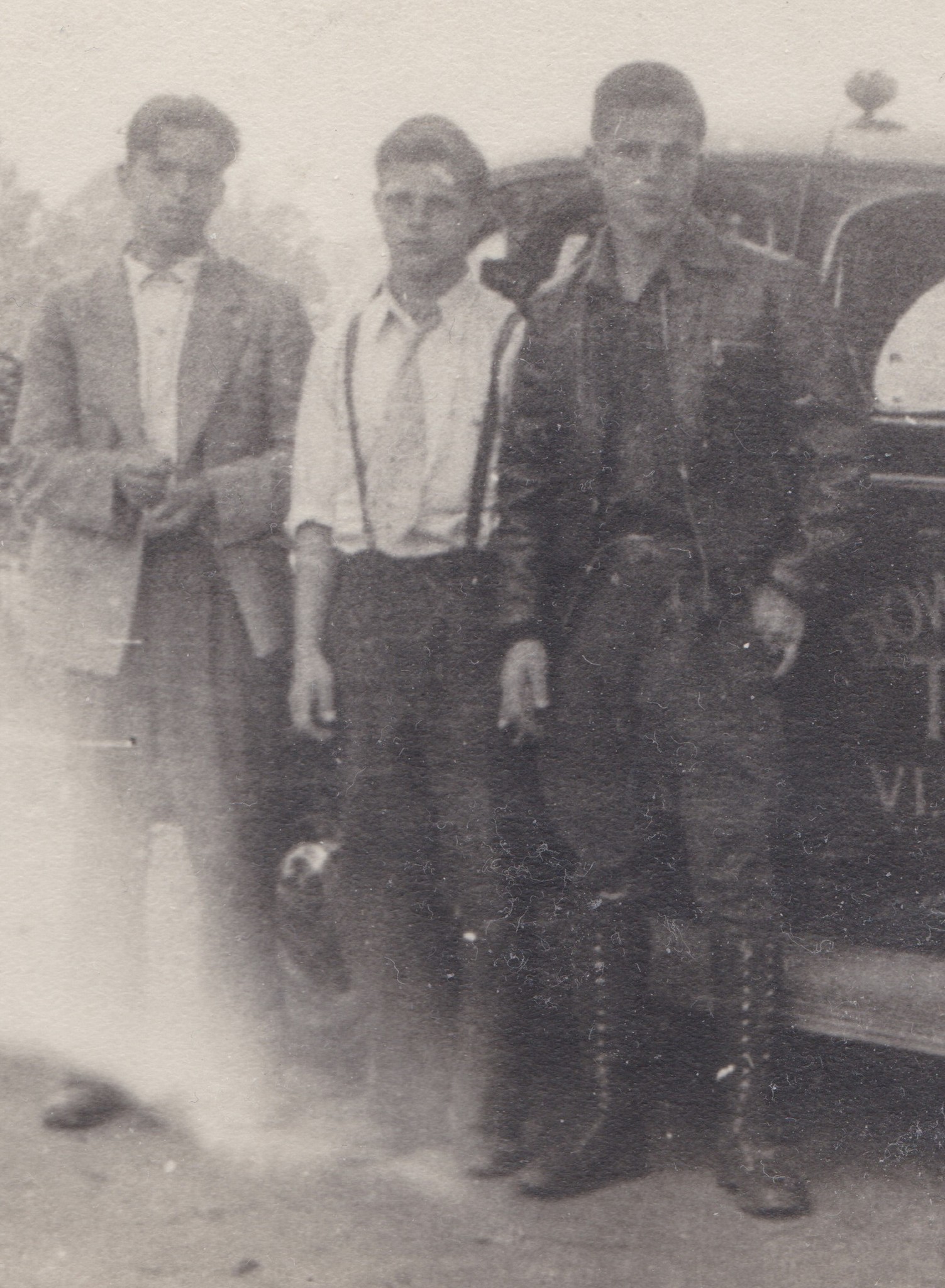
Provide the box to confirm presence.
[366,309,428,543]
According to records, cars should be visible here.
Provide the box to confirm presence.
[262,139,942,1058]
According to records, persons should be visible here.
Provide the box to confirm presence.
[7,92,307,1130]
[494,62,871,1219]
[284,113,539,1180]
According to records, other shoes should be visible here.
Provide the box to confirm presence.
[39,1079,122,1129]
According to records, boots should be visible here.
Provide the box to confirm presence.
[704,917,812,1217]
[330,897,437,1166]
[518,914,649,1197]
[448,905,523,1177]
